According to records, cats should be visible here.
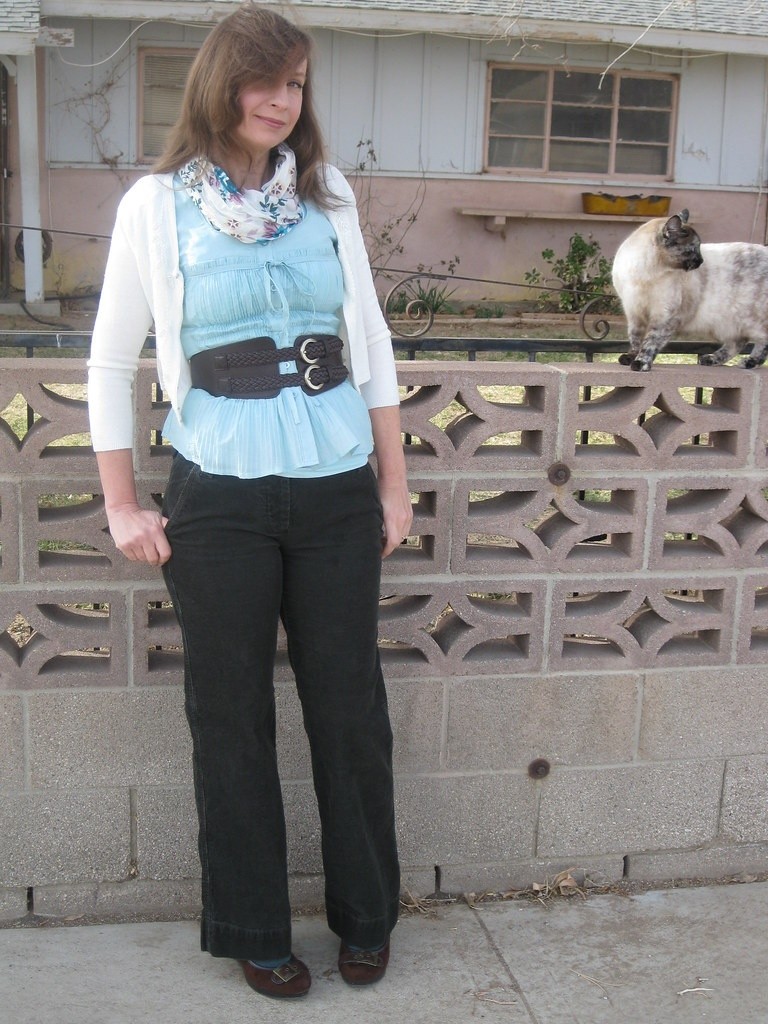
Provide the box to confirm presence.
[612,208,768,372]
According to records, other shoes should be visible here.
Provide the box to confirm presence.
[237,955,311,998]
[337,932,390,986]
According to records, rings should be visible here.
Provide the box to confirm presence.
[401,537,405,539]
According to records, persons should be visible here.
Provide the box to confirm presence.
[87,10,414,997]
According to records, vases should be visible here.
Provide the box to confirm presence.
[580,192,671,216]
